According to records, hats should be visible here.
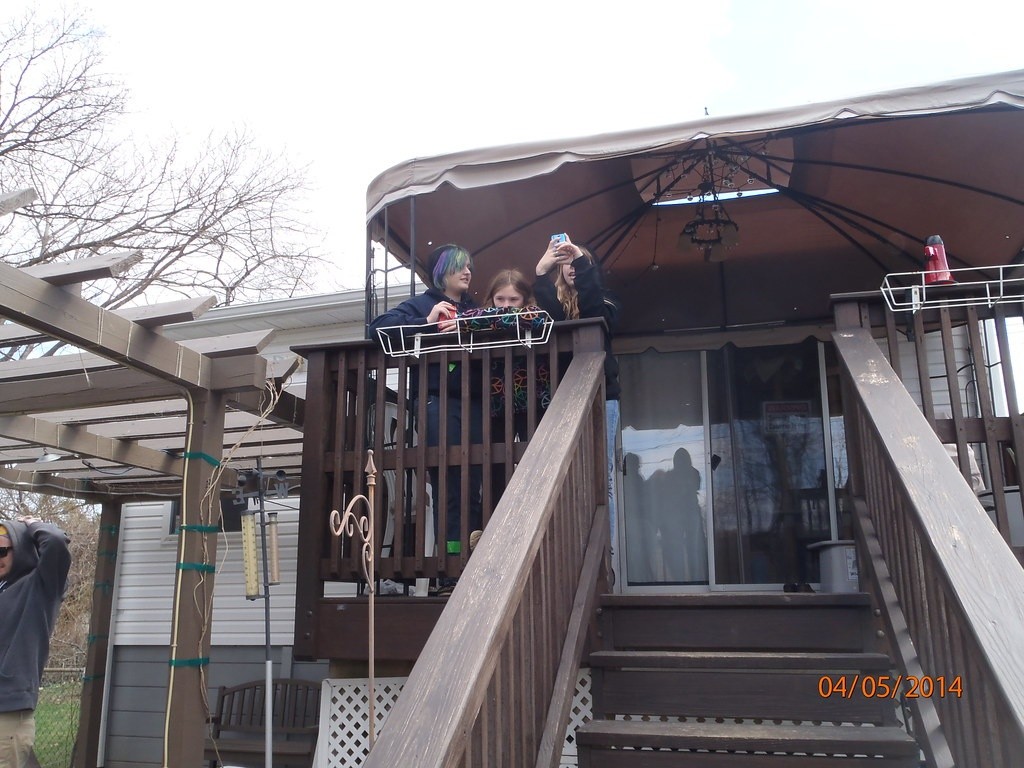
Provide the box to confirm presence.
[427,244,462,286]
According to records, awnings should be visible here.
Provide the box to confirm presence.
[354,67,1024,333]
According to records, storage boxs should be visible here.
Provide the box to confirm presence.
[807,541,860,593]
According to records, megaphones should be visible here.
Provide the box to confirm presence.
[923,235,958,285]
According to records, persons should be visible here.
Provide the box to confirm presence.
[0,515,72,768]
[439,267,550,554]
[531,233,618,586]
[366,243,492,594]
[623,447,708,584]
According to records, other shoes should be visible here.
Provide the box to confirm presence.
[470,530,482,553]
[363,578,416,596]
[436,582,456,595]
[783,579,815,593]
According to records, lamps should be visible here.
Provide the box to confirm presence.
[241,509,280,601]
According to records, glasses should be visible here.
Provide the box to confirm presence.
[0,547,13,558]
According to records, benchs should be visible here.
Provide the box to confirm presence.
[204,679,322,768]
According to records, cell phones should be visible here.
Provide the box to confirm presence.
[551,233,566,246]
[438,306,455,329]
